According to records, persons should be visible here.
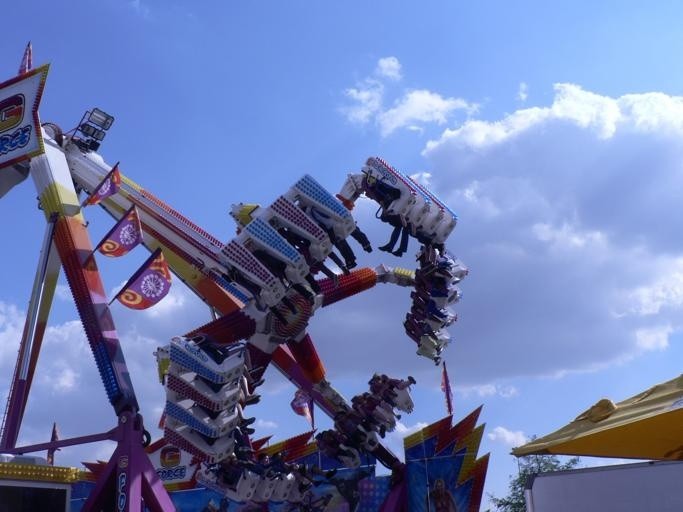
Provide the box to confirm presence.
[187,173,463,511]
[425,476,458,511]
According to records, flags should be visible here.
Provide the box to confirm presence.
[81,166,123,211]
[95,205,145,261]
[114,248,174,311]
